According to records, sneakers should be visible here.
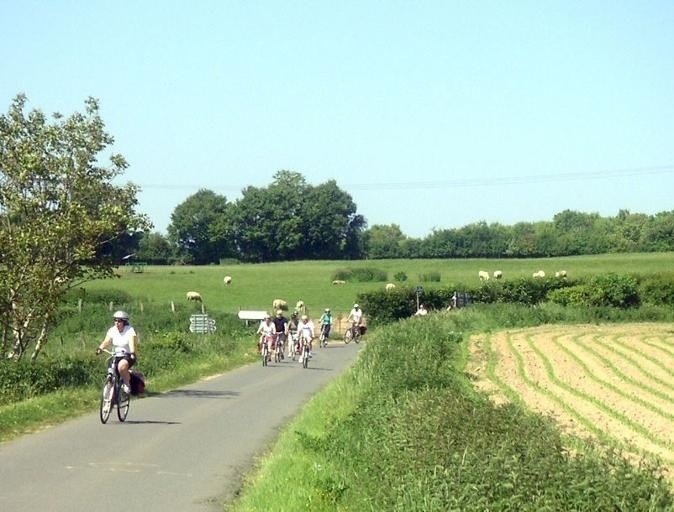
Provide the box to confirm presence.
[102,402,110,412]
[260,348,312,363]
[123,384,131,394]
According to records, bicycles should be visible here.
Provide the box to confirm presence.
[343,319,362,344]
[318,322,332,348]
[96,347,130,423]
[257,329,313,369]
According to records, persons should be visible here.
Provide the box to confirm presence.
[318,307,333,348]
[256,316,277,357]
[346,303,363,340]
[287,311,299,359]
[271,309,289,363]
[414,302,428,317]
[296,314,316,358]
[95,310,139,414]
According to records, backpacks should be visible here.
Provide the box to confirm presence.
[129,372,144,395]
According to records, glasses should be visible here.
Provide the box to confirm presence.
[114,318,122,322]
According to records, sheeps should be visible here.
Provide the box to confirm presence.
[186,291,202,304]
[532,270,545,280]
[478,270,490,282]
[493,271,503,281]
[385,282,395,289]
[223,276,232,287]
[294,300,305,311]
[332,280,346,286]
[554,271,567,279]
[272,298,288,311]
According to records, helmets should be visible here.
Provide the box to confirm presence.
[261,303,359,322]
[112,311,129,320]
